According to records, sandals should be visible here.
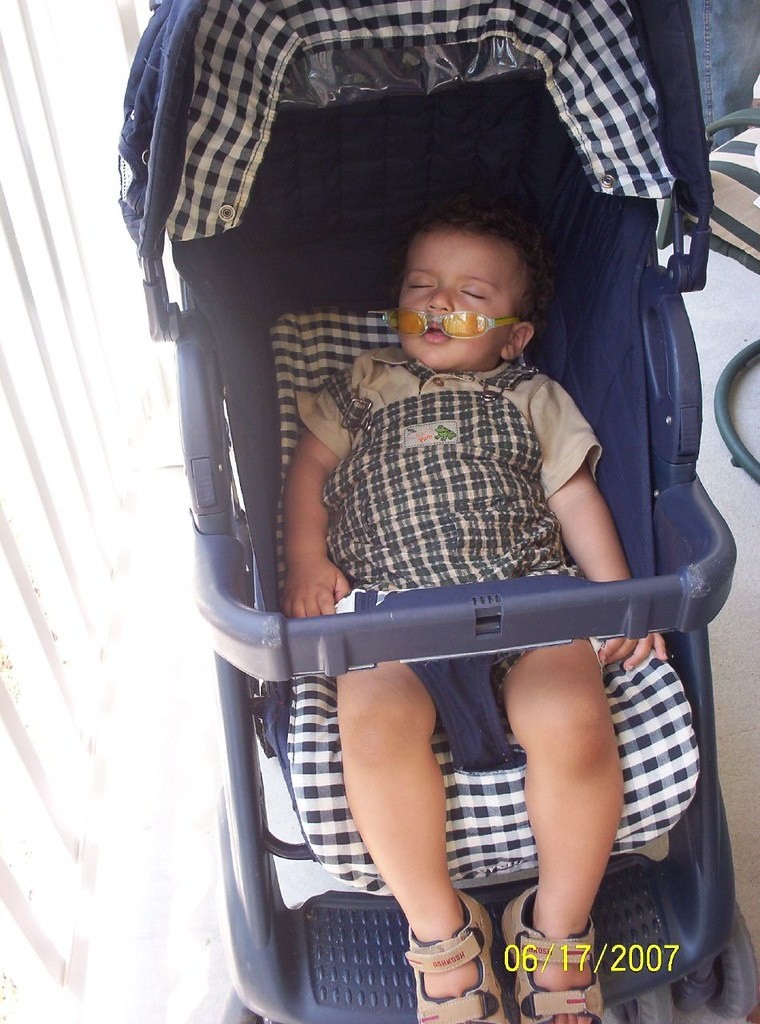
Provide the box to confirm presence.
[405,890,505,1024]
[501,886,603,1024]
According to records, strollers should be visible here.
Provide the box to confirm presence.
[110,1,759,1024]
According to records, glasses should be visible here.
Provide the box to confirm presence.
[383,307,520,340]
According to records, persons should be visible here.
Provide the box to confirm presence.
[279,210,668,1024]
